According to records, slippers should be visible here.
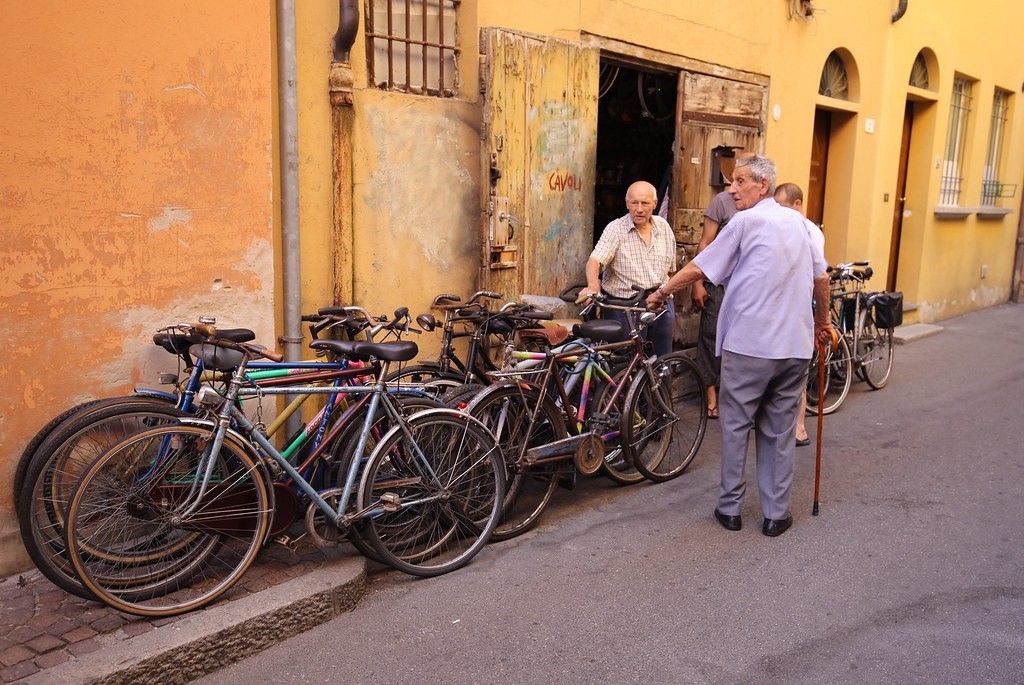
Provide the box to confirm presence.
[708,406,719,419]
[795,438,811,447]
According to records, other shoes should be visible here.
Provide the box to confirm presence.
[714,507,742,531]
[763,512,793,536]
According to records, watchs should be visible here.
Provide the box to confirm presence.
[658,283,673,297]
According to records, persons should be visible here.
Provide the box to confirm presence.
[578,180,676,442]
[773,182,812,447]
[691,189,741,419]
[646,153,833,535]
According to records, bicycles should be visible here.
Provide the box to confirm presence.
[806,258,905,419]
[17,283,713,619]
